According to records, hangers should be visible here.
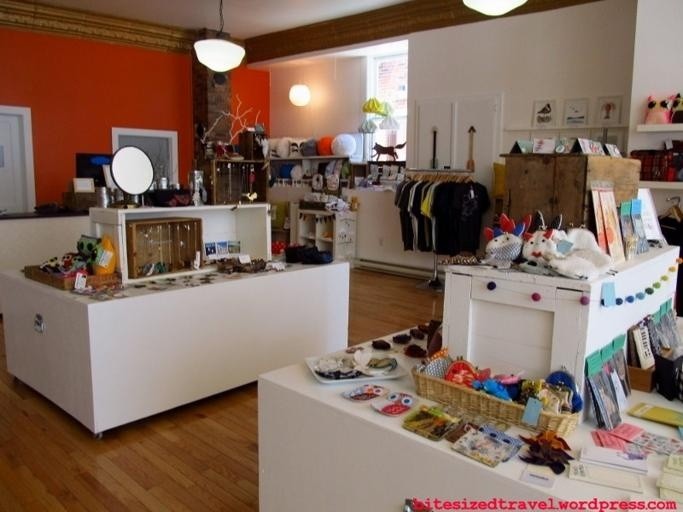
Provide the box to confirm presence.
[658,196,682,223]
[404,167,477,183]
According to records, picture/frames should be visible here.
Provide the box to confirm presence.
[562,98,589,125]
[595,95,621,125]
[531,99,556,128]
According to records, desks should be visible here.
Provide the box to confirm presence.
[1,202,349,442]
[258,248,683,511]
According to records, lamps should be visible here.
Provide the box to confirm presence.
[288,83,310,106]
[463,0,528,16]
[194,2,246,73]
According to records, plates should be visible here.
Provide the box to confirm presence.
[372,392,419,418]
[340,384,390,402]
[303,350,408,383]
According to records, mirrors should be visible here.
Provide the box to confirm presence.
[111,145,155,207]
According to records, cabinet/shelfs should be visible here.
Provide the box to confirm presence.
[290,201,358,269]
[211,159,267,204]
[270,155,350,243]
[635,123,683,191]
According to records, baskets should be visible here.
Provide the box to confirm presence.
[24,265,118,290]
[411,354,579,437]
[63,192,96,212]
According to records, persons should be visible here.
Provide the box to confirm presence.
[644,94,676,124]
